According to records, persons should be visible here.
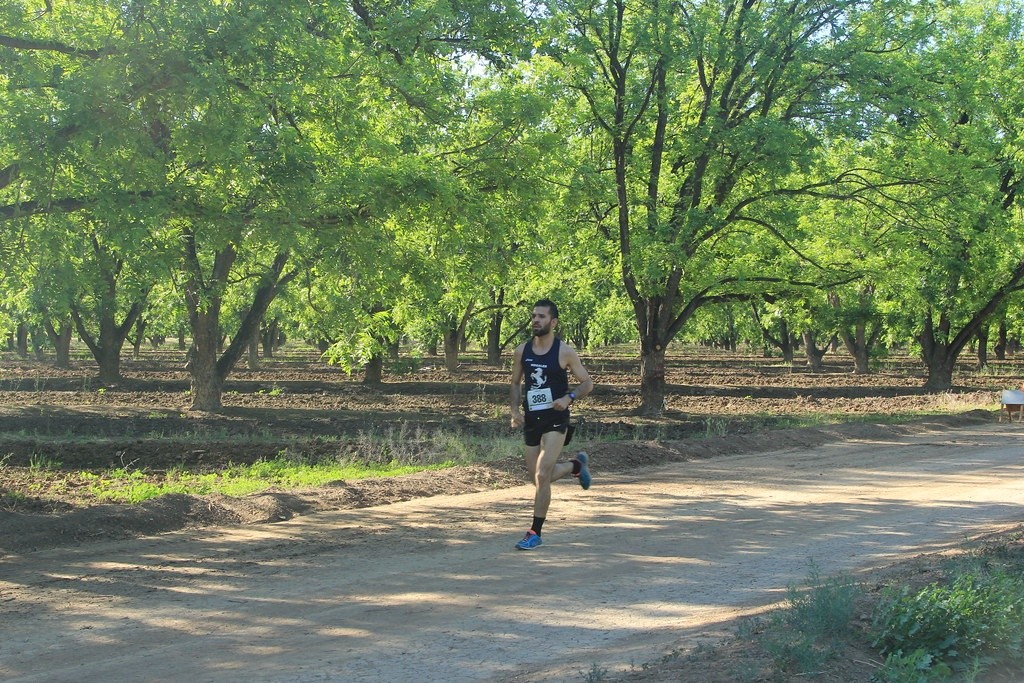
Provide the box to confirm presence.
[510,299,593,550]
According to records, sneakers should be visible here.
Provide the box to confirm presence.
[514,530,544,549]
[575,451,592,490]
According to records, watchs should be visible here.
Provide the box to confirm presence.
[569,393,576,403]
